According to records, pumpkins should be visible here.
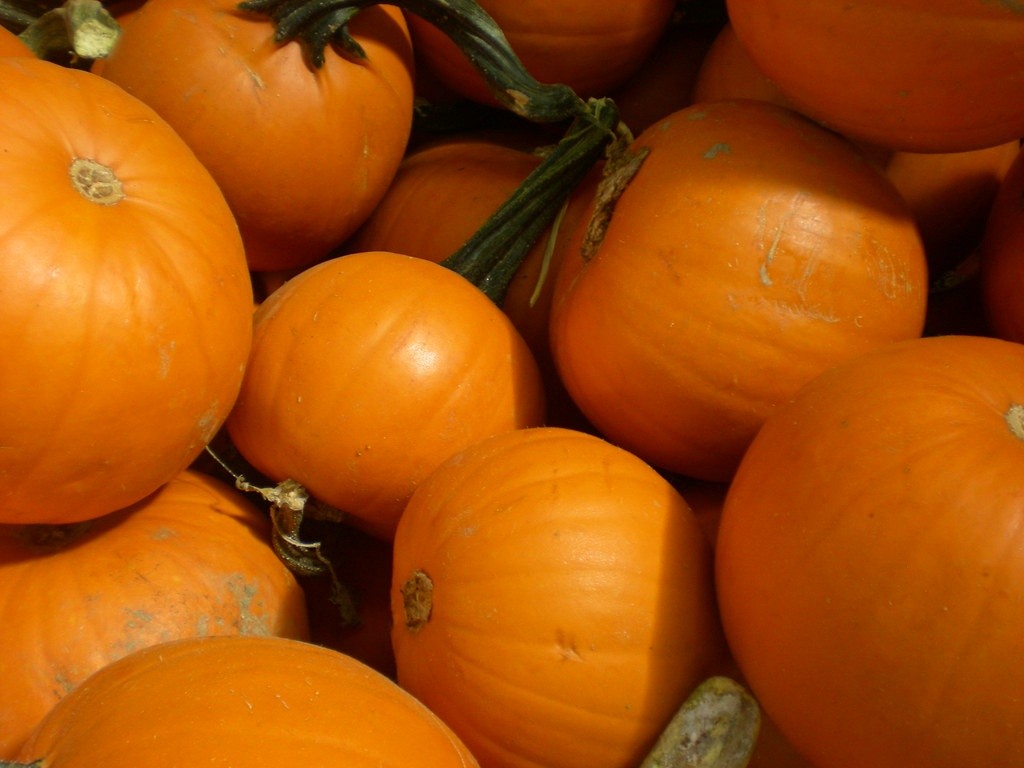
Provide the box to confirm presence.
[0,0,1024,768]
